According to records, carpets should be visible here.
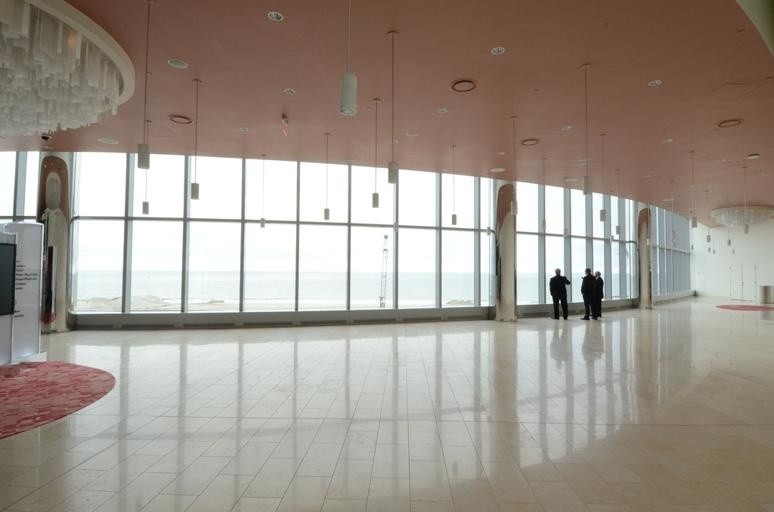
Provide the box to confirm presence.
[1,360,116,438]
[716,305,773,311]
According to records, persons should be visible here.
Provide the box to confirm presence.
[591,272,603,317]
[549,269,571,320]
[581,268,598,320]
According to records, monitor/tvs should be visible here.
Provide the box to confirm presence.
[0,243,17,315]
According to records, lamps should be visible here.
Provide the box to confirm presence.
[141,120,148,216]
[615,170,620,234]
[191,80,199,198]
[670,195,675,240]
[600,136,606,222]
[584,68,592,195]
[646,199,651,246]
[487,228,492,235]
[260,156,265,227]
[452,145,456,225]
[690,188,736,255]
[691,156,696,228]
[744,158,748,234]
[373,99,378,209]
[139,3,152,171]
[323,133,329,219]
[511,117,517,216]
[389,34,397,184]
